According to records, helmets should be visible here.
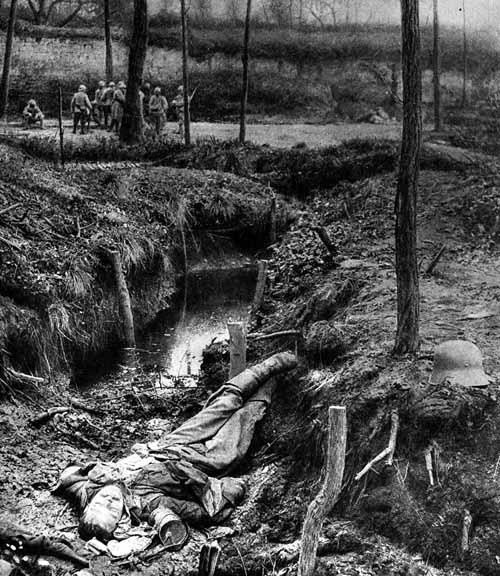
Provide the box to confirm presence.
[75,78,187,95]
[427,338,491,387]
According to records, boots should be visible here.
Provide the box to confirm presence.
[69,117,185,136]
[226,352,306,406]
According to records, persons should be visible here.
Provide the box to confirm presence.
[46,350,299,563]
[20,99,47,131]
[67,78,193,136]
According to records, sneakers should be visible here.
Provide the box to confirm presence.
[21,126,47,131]
